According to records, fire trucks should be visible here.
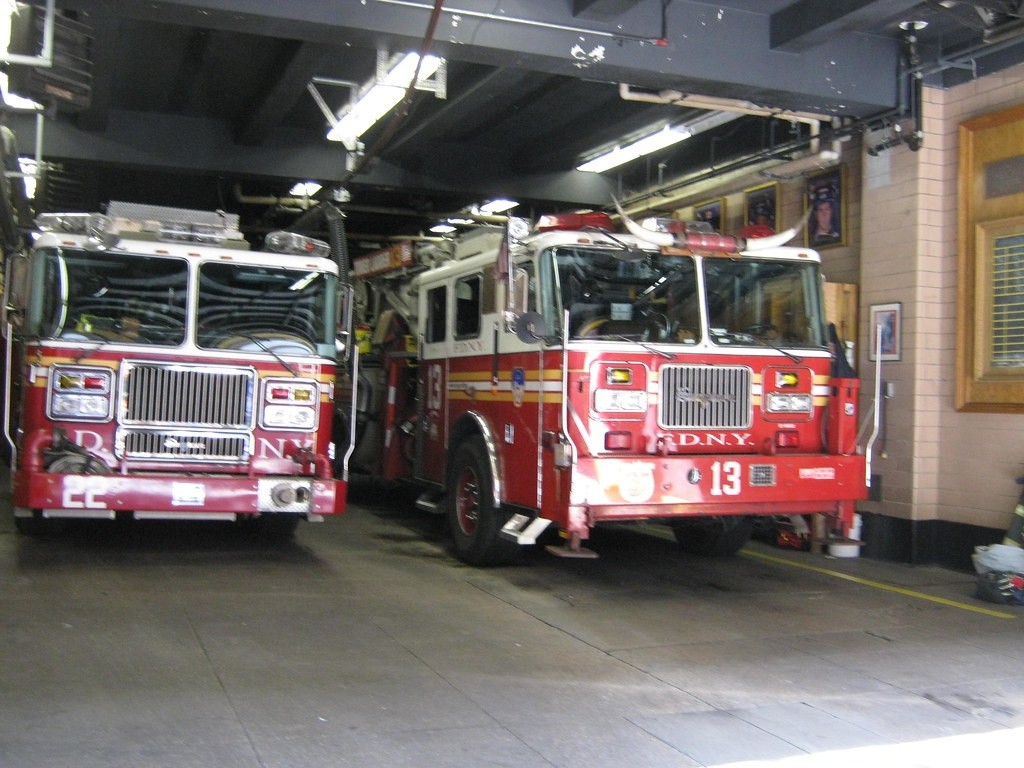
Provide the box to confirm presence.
[2,199,362,540]
[340,210,884,570]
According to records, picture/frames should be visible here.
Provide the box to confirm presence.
[803,162,846,252]
[868,302,902,362]
[661,212,679,220]
[693,198,726,234]
[744,180,781,234]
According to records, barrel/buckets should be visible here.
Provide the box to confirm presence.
[828,514,862,557]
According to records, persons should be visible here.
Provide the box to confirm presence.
[809,182,840,248]
[749,192,775,227]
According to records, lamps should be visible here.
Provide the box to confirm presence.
[328,51,444,142]
[575,109,744,173]
